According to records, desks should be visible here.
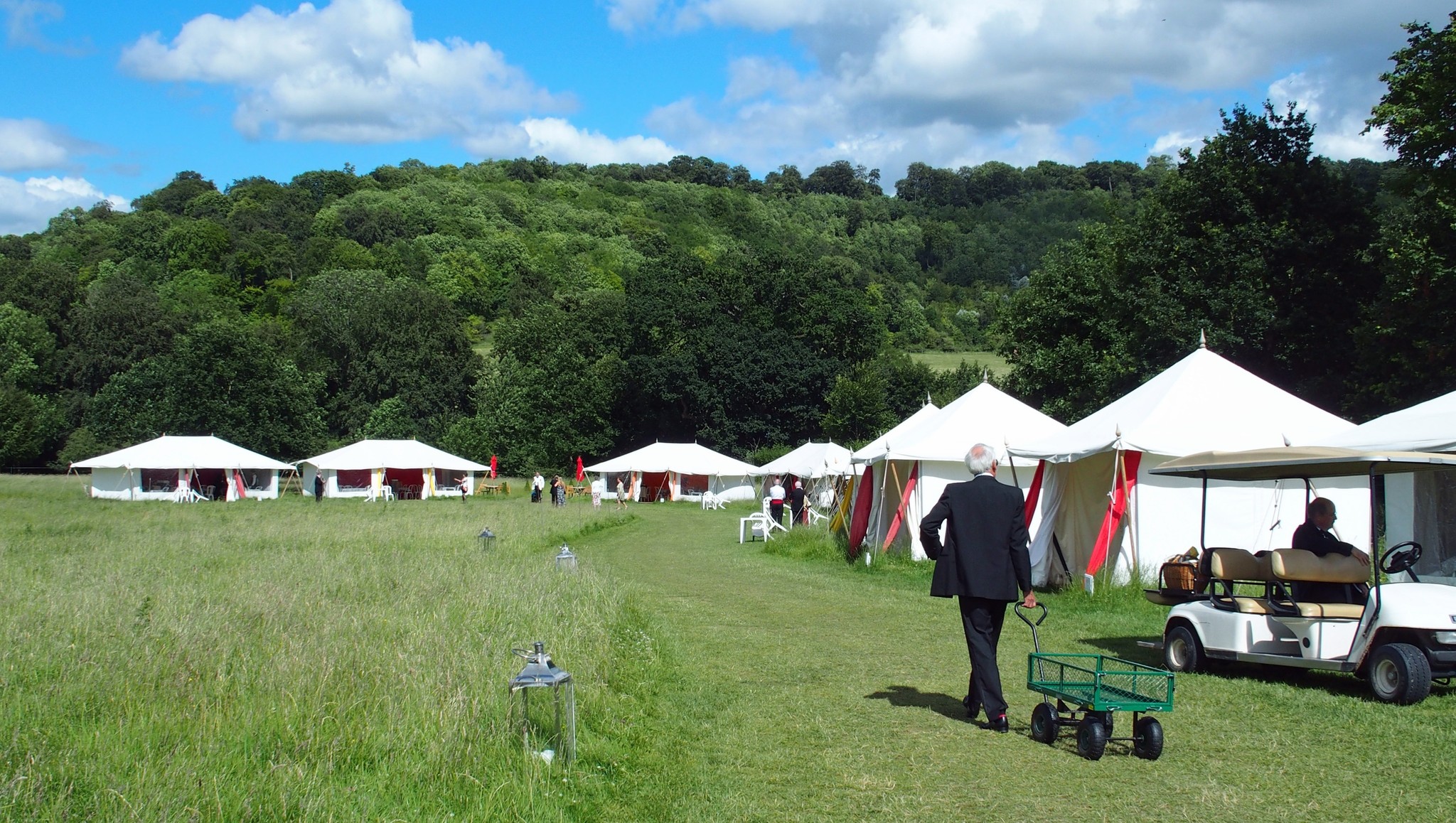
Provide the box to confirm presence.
[700,496,714,509]
[740,517,768,544]
[340,488,366,492]
[440,487,455,491]
[372,488,387,503]
[484,485,498,494]
[571,487,585,497]
[691,492,703,496]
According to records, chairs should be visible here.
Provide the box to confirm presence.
[244,486,263,491]
[749,497,830,542]
[143,485,226,503]
[475,483,511,495]
[435,484,461,491]
[680,487,731,511]
[565,485,591,498]
[639,486,670,501]
[338,485,353,489]
[364,484,423,502]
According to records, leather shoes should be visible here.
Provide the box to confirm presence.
[984,714,1010,734]
[963,694,980,718]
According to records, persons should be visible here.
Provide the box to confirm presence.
[530,471,567,508]
[454,472,468,503]
[247,467,324,502]
[615,477,628,509]
[769,479,809,533]
[919,444,1036,733]
[591,475,604,512]
[1290,497,1369,606]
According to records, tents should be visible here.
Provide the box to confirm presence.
[290,435,492,498]
[746,435,850,510]
[823,367,1068,562]
[1317,389,1456,590]
[1003,328,1375,592]
[70,433,298,501]
[583,438,760,503]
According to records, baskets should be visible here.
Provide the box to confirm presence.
[1163,555,1198,590]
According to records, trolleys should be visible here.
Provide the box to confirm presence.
[1013,600,1176,762]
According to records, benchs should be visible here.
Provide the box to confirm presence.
[1199,548,1372,619]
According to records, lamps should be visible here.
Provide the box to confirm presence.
[555,542,579,570]
[507,642,575,769]
[478,527,497,552]
